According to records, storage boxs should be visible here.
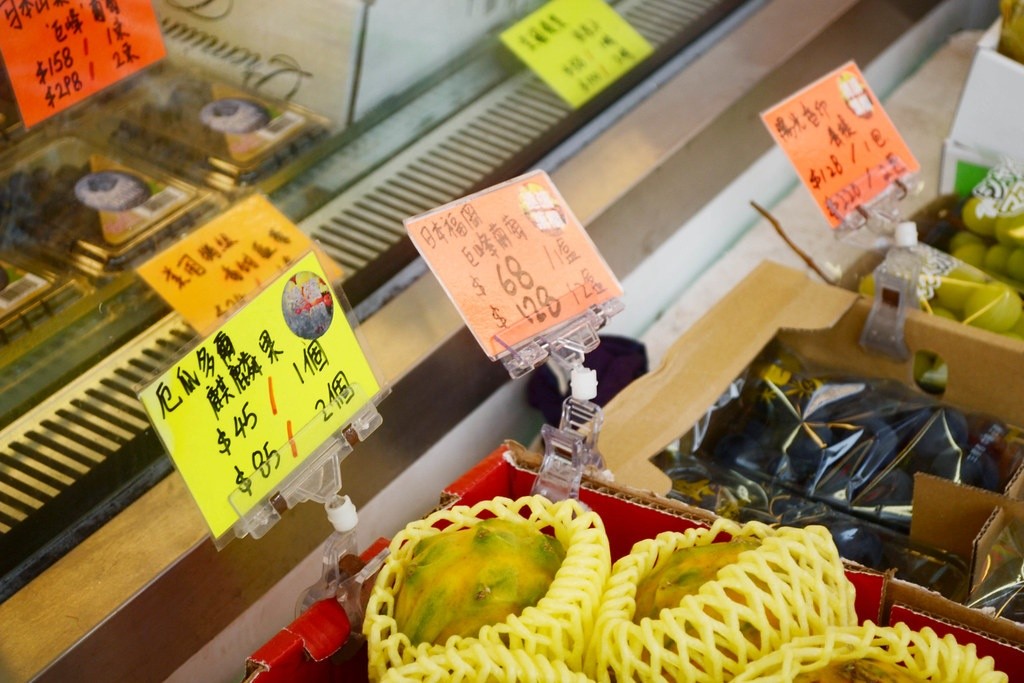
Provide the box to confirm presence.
[237,16,1024,683]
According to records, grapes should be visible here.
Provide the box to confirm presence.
[860,194,1024,391]
[660,371,1024,627]
